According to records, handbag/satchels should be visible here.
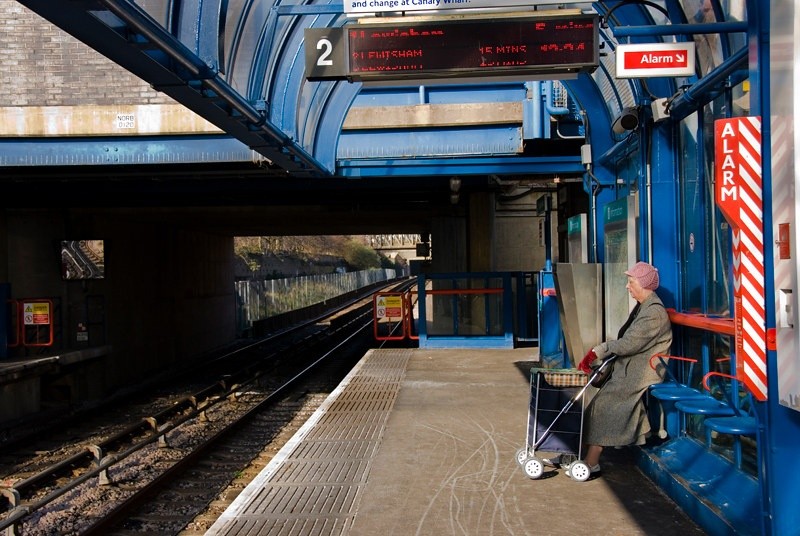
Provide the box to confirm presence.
[589,360,614,388]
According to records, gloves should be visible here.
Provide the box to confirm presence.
[577,348,597,373]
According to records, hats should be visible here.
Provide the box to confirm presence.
[624,262,659,290]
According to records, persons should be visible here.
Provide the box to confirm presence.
[62,259,70,279]
[542,262,674,478]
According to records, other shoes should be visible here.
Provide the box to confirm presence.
[542,457,569,470]
[565,463,601,476]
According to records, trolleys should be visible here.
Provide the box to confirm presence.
[515,353,617,483]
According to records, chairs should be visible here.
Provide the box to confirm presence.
[649,353,763,470]
